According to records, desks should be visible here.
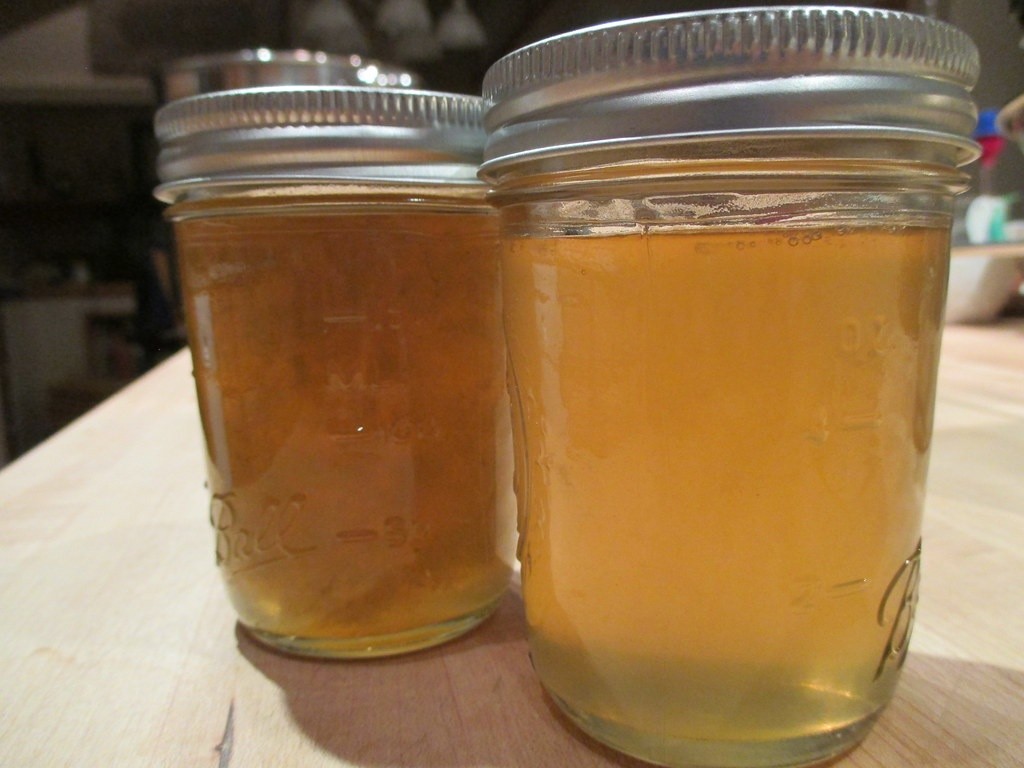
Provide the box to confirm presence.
[0,321,1024,768]
